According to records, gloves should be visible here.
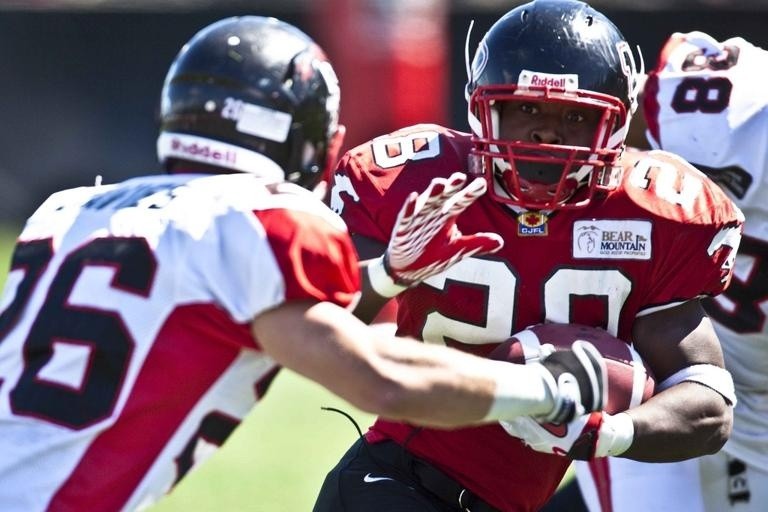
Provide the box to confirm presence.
[499,340,636,462]
[369,172,505,299]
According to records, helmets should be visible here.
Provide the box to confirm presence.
[465,1,639,211]
[156,15,340,191]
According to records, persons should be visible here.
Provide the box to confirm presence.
[624,30,767,510]
[1,16,610,511]
[308,1,745,511]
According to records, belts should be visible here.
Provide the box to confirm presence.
[373,439,495,511]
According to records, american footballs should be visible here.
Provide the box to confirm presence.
[489,324,656,414]
